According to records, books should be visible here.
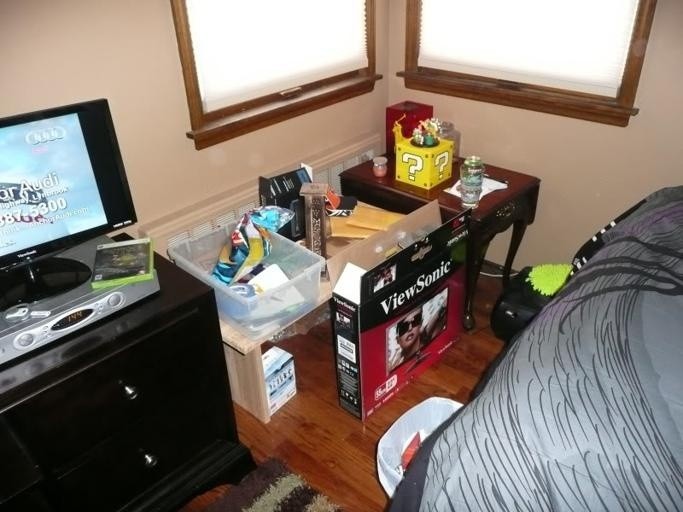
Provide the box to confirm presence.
[90,237,154,287]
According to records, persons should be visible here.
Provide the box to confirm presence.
[394,299,447,363]
[383,267,393,287]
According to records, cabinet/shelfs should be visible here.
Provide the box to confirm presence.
[0,232,257,512]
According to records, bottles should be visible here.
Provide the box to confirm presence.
[459,156,485,210]
[372,156,387,178]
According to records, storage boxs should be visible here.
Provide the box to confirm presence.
[168,220,326,341]
[326,198,472,421]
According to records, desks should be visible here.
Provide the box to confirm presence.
[219,233,366,424]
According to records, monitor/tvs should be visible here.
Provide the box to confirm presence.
[0,98,138,313]
[386,286,449,376]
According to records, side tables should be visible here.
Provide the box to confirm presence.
[339,153,541,331]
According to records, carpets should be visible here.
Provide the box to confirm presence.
[204,459,344,512]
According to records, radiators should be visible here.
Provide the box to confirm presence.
[138,130,383,263]
